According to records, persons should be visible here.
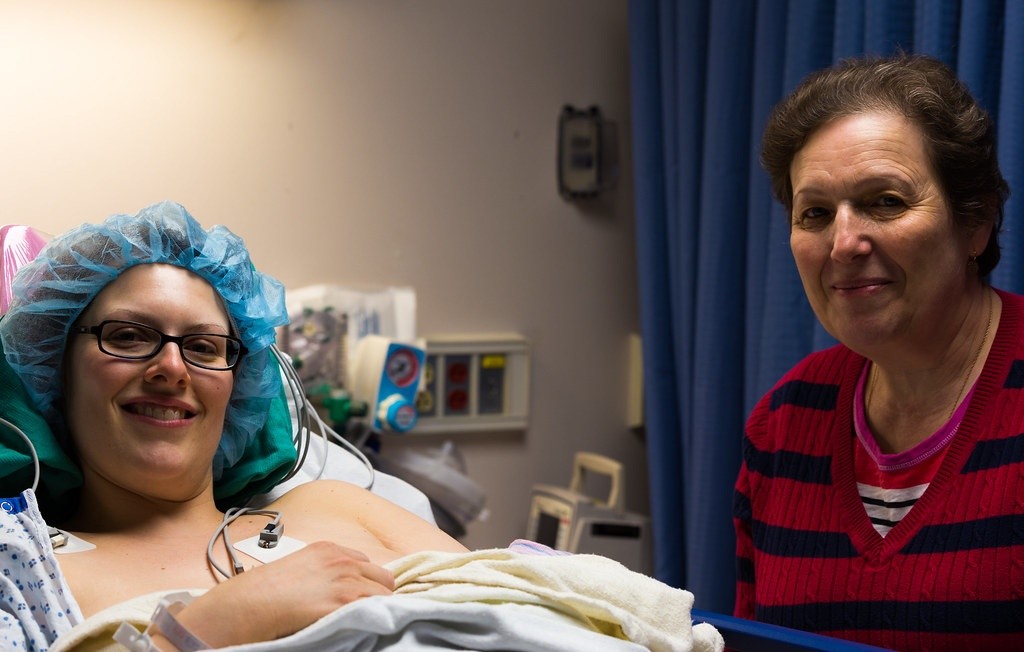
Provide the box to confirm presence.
[729,56,1024,652]
[0,200,471,652]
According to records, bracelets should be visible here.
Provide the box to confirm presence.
[112,598,213,652]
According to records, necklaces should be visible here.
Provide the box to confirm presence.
[864,286,992,419]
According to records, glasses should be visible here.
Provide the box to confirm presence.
[69,318,250,372]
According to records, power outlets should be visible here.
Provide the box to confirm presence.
[408,333,531,433]
